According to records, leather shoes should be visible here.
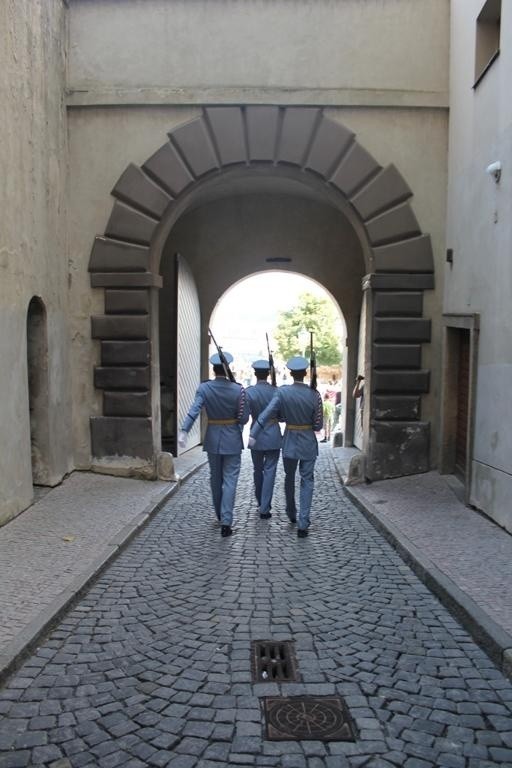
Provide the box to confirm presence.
[298,529,308,538]
[221,526,232,537]
[261,512,272,519]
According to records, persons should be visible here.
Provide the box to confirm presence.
[176,353,323,538]
[306,364,364,443]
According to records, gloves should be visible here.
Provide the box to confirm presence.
[247,437,256,449]
[178,432,188,448]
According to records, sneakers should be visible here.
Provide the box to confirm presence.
[320,439,327,443]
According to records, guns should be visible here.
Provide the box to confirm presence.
[208,329,244,382]
[266,333,276,386]
[310,332,317,389]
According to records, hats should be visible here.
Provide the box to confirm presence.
[286,356,309,375]
[210,352,233,367]
[252,359,271,372]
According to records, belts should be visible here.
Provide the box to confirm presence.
[286,425,312,430]
[208,420,237,425]
[251,419,278,424]
